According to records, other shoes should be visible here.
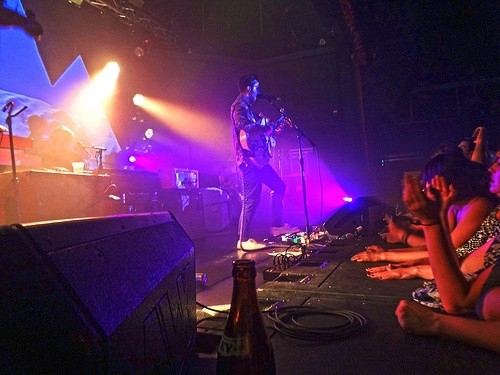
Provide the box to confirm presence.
[236,237,266,250]
[269,224,299,235]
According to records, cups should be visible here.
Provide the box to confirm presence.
[72,162,85,174]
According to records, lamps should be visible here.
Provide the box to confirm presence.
[134,41,149,58]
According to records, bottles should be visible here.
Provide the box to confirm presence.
[217,259,275,375]
[339,226,364,241]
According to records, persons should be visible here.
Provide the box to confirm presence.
[47,125,85,171]
[230,74,300,250]
[351,127,500,351]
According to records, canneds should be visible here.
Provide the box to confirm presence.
[195,273,207,285]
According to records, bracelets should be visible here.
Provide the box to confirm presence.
[402,231,411,246]
[421,220,441,226]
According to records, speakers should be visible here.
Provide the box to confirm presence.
[323,197,384,236]
[0,169,206,375]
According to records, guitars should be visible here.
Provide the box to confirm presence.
[240,110,288,169]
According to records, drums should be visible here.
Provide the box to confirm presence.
[199,184,243,233]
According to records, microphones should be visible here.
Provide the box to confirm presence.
[92,146,107,151]
[256,94,281,100]
